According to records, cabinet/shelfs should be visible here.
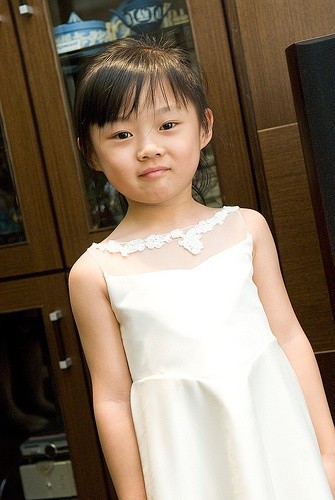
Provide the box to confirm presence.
[0,0,335,500]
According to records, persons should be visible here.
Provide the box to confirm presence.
[69,38,335,500]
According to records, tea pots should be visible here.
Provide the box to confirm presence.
[109,0,171,33]
